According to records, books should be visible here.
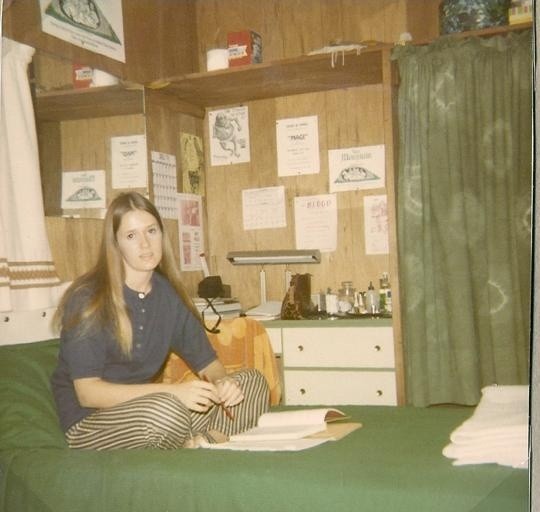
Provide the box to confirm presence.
[191,296,242,320]
[231,407,345,442]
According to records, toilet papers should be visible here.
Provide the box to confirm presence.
[325,294,338,314]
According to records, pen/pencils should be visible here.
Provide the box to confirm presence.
[203,374,235,422]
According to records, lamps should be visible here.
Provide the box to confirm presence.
[227,249,321,321]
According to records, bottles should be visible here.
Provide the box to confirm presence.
[338,282,355,308]
[368,281,377,312]
[379,272,392,313]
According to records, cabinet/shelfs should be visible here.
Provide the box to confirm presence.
[264,320,396,407]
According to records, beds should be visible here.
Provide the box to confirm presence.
[1,337,530,512]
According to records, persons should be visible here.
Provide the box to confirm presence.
[48,190,272,454]
